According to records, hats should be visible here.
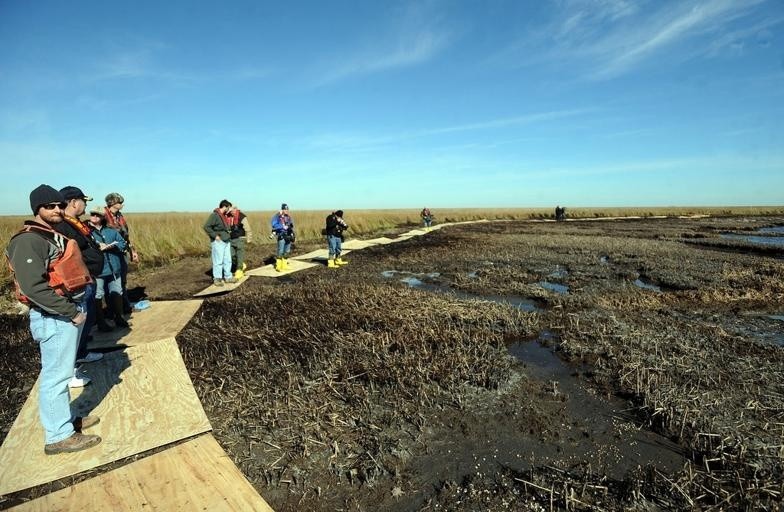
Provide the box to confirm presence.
[335,210,343,218]
[59,186,93,201]
[90,205,107,217]
[281,204,289,210]
[29,183,68,216]
[105,193,124,207]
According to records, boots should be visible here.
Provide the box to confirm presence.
[282,257,287,271]
[335,258,348,265]
[275,258,281,272]
[328,259,339,268]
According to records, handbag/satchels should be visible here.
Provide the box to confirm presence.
[81,246,104,277]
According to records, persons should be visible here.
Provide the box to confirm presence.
[85,206,128,332]
[271,203,294,272]
[555,205,566,222]
[102,193,138,314]
[325,210,348,269]
[56,187,102,386]
[225,204,251,280]
[421,207,433,227]
[4,183,102,455]
[204,199,238,286]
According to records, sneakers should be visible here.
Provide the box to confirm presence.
[114,317,129,328]
[77,352,105,363]
[44,433,102,455]
[97,322,113,333]
[213,262,247,287]
[72,416,100,431]
[68,368,92,388]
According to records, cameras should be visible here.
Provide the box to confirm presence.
[269,230,279,240]
[337,217,347,231]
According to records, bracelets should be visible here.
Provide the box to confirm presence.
[225,212,228,215]
